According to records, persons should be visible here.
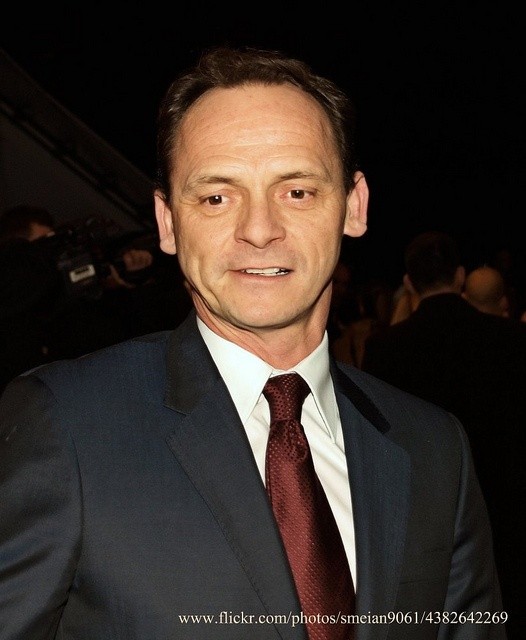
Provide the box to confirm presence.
[0,48,509,634]
[1,204,522,632]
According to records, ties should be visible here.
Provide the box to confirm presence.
[263,373,355,635]
